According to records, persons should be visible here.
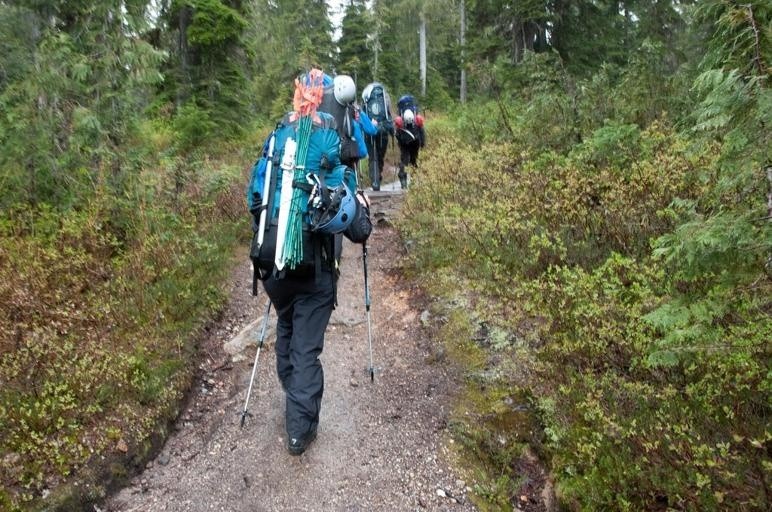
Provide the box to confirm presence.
[339,81,423,191]
[248,68,373,455]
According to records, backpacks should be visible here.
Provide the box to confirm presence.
[245,112,356,283]
[362,83,393,135]
[396,95,422,146]
[293,68,358,161]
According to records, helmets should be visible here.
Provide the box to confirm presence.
[309,182,356,233]
[334,75,356,106]
[403,110,414,124]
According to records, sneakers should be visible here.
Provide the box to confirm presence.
[289,427,317,454]
